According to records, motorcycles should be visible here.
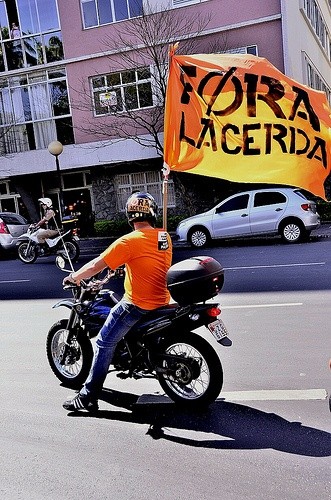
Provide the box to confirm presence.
[46,255,233,409]
[17,219,80,265]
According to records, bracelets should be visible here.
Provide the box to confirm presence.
[70,273,76,282]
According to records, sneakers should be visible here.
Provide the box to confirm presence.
[62,392,99,413]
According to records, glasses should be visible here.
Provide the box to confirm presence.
[42,204,47,206]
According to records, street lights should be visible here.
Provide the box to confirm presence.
[47,140,66,217]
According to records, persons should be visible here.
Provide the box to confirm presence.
[63,192,172,411]
[10,22,21,46]
[31,198,61,252]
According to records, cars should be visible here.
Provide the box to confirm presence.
[0,211,30,262]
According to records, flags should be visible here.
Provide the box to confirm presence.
[163,53,331,201]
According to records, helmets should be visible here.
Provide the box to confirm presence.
[125,192,159,228]
[38,197,53,208]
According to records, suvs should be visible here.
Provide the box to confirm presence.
[175,188,321,249]
